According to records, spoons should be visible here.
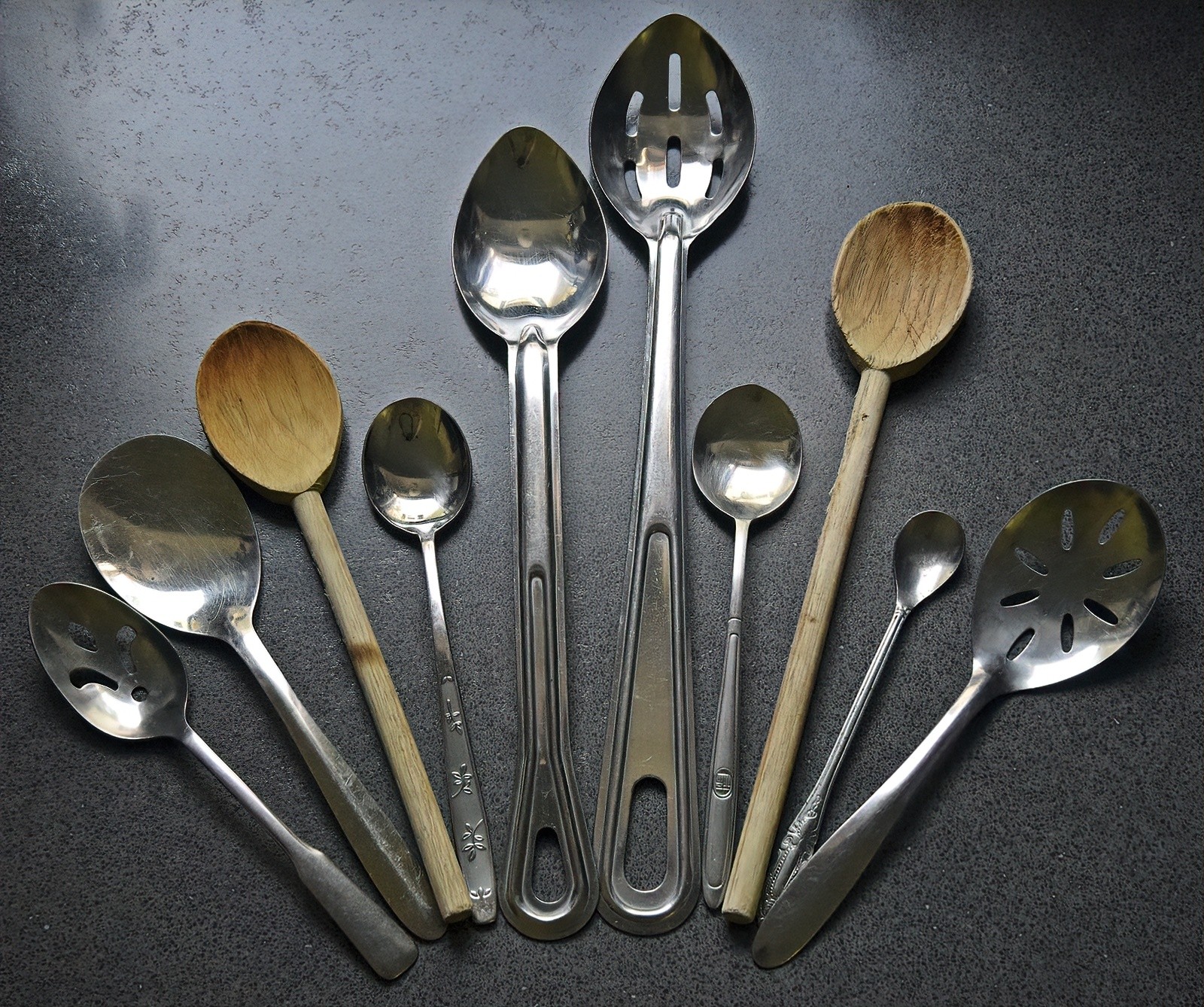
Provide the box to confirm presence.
[199,321,475,924]
[451,125,600,939]
[29,582,417,983]
[79,434,450,942]
[714,202,1163,924]
[362,397,499,926]
[691,383,805,912]
